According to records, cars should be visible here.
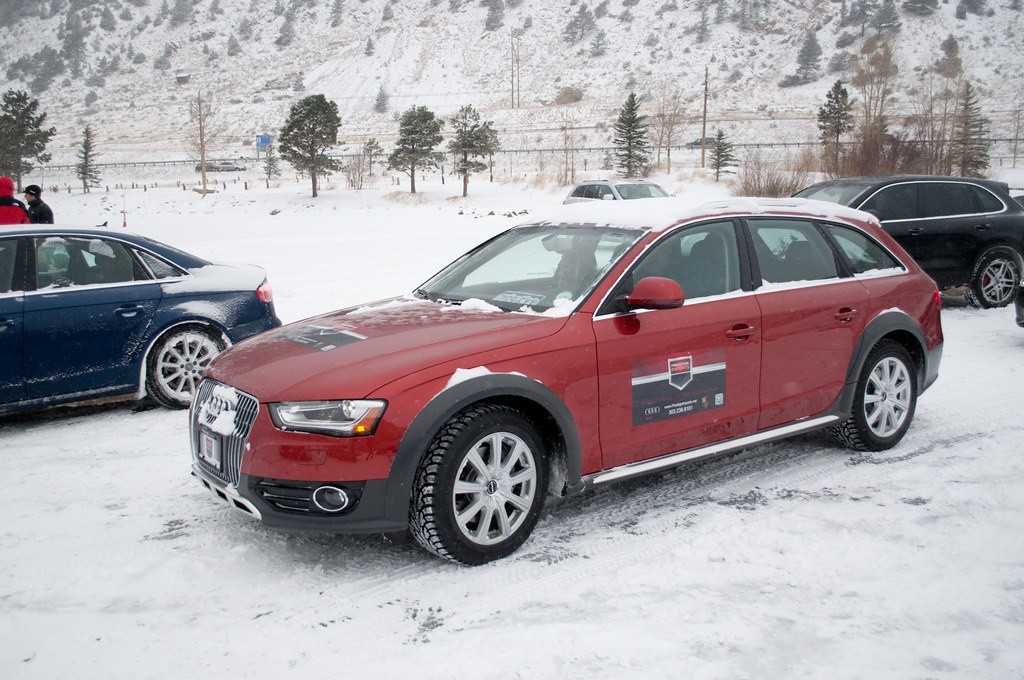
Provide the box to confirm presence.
[189,194,943,566]
[0,224,284,419]
[195,162,222,172]
[682,174,1024,309]
[563,177,669,200]
[686,138,715,150]
[218,161,246,172]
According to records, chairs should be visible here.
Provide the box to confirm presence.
[634,231,731,298]
[780,241,825,280]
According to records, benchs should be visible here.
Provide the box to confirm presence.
[62,242,131,283]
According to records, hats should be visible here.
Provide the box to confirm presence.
[23,185,41,197]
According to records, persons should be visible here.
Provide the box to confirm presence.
[0,176,31,224]
[23,185,54,224]
